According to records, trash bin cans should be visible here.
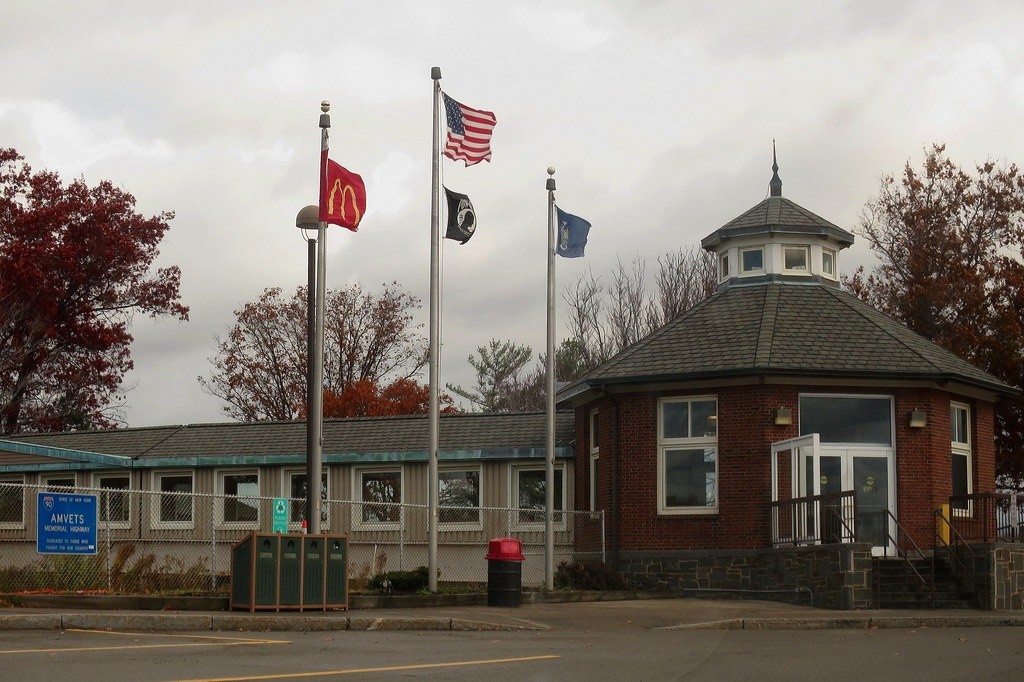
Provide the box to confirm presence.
[485,538,525,607]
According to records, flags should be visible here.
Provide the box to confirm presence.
[319,149,367,232]
[443,185,477,245]
[557,207,592,258]
[441,91,497,168]
